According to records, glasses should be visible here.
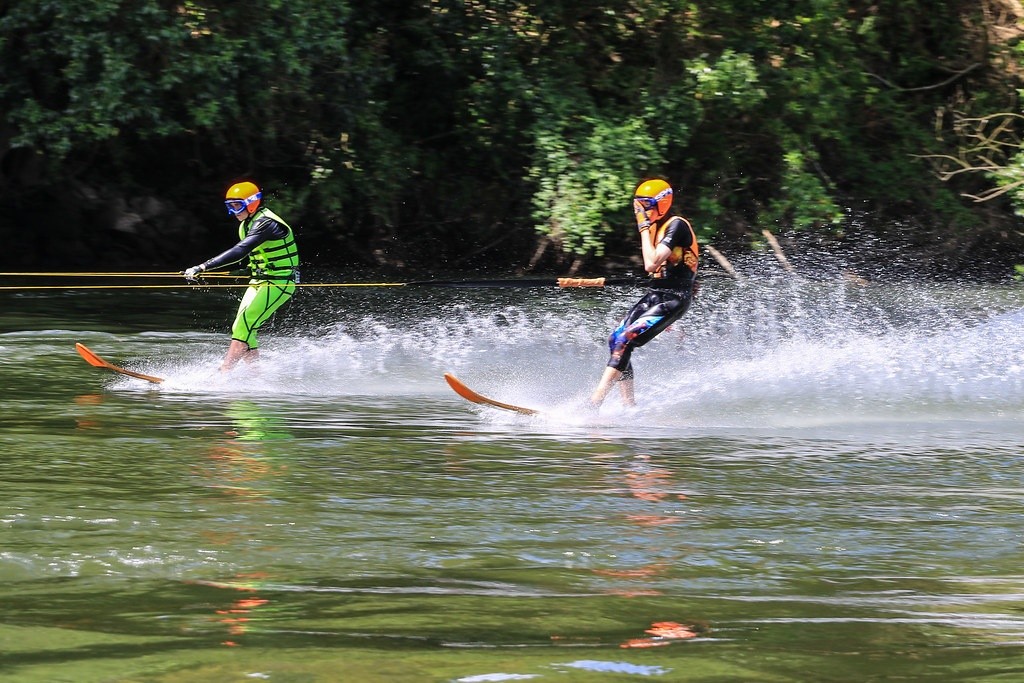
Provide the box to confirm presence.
[636,198,656,211]
[225,200,246,214]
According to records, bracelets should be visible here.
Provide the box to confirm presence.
[199,264,205,271]
[639,227,648,232]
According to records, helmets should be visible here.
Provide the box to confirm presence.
[225,182,260,213]
[635,180,672,215]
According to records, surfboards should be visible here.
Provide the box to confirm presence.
[444,373,540,417]
[75,341,167,385]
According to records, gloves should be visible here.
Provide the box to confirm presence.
[185,265,203,283]
[234,266,252,284]
[632,199,650,232]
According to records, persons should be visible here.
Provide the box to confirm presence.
[185,182,299,373]
[583,179,699,419]
